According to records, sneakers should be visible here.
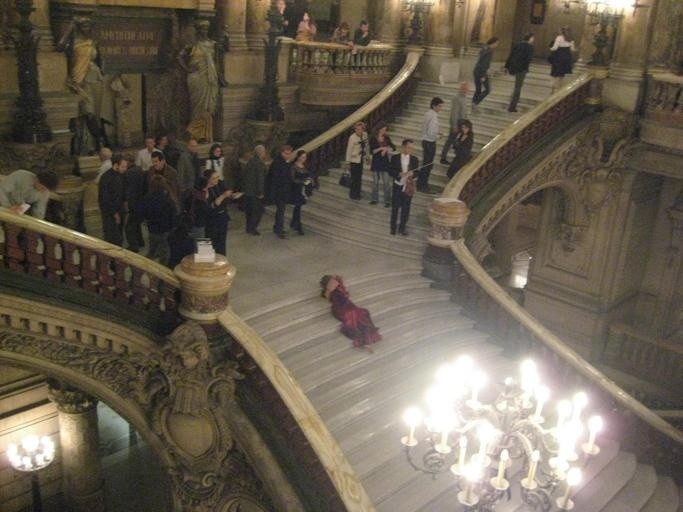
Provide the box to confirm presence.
[440,159,449,164]
[369,200,390,207]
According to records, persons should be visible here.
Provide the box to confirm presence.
[327,22,353,72]
[176,19,226,143]
[294,11,316,67]
[388,139,418,235]
[446,121,473,180]
[547,25,579,94]
[59,15,102,116]
[471,37,499,114]
[439,81,471,164]
[345,122,369,201]
[93,134,317,312]
[351,20,370,72]
[0,167,57,274]
[416,97,444,193]
[273,0,287,36]
[368,121,395,207]
[504,33,534,112]
[318,274,382,353]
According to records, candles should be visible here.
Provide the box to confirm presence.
[405,363,607,503]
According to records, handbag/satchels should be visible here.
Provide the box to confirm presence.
[340,177,353,188]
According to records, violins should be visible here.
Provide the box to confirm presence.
[404,178,414,198]
